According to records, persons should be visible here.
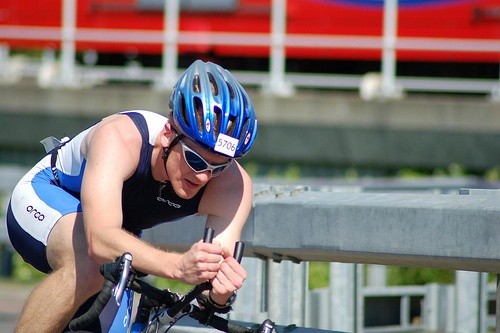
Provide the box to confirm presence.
[6,58,260,333]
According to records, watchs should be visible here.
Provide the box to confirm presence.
[207,290,238,309]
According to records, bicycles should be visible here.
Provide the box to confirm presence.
[60,250,296,333]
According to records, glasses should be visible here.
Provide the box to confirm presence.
[168,121,234,178]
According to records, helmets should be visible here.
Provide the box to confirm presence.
[170,59,258,159]
